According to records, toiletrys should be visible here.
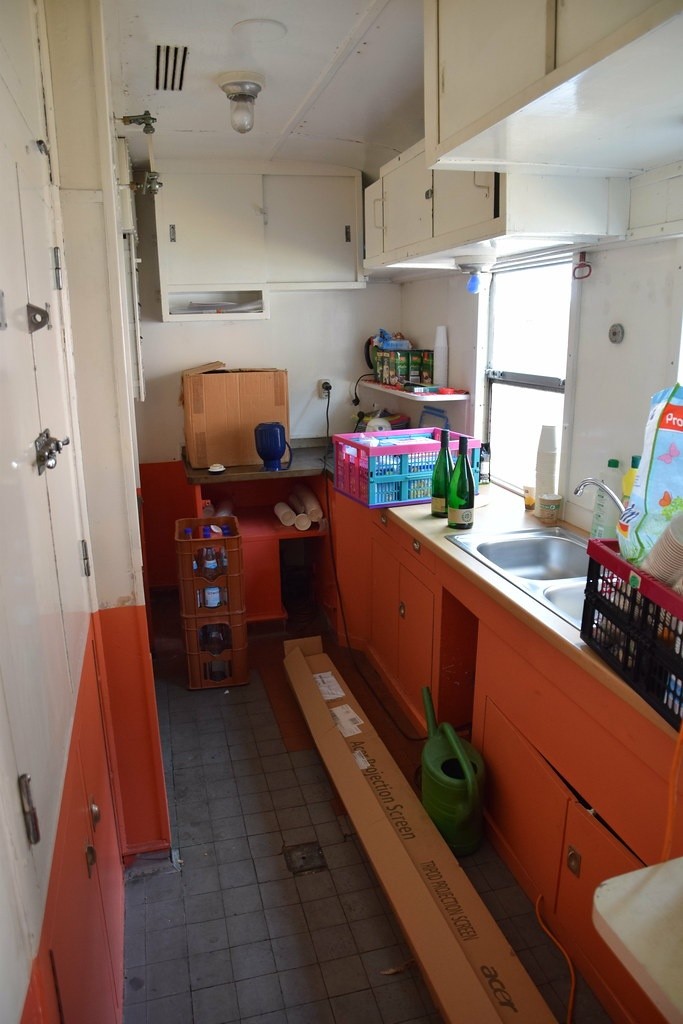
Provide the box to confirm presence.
[589,459,624,539]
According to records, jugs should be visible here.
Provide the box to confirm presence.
[419,686,487,856]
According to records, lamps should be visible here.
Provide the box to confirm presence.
[218,71,263,133]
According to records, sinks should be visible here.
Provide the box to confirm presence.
[533,578,624,631]
[444,526,625,589]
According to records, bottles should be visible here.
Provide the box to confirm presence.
[199,627,232,682]
[446,436,475,530]
[431,429,455,518]
[590,455,642,540]
[182,525,230,607]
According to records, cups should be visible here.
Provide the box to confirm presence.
[598,503,683,665]
[274,483,323,531]
[533,424,563,527]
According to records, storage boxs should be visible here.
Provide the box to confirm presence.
[332,423,481,508]
[580,538,683,737]
[175,517,251,690]
[182,360,292,470]
[283,635,557,1024]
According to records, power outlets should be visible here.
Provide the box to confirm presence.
[321,379,330,397]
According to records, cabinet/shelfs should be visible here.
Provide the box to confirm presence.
[424,0,683,176]
[360,177,384,256]
[370,513,444,727]
[471,626,682,1023]
[384,152,432,250]
[194,484,331,625]
[153,167,363,322]
[432,168,500,237]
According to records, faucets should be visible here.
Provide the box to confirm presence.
[573,477,626,515]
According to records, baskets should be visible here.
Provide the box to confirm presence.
[581,539,683,733]
[331,428,481,509]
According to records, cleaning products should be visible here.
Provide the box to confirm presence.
[619,454,642,520]
